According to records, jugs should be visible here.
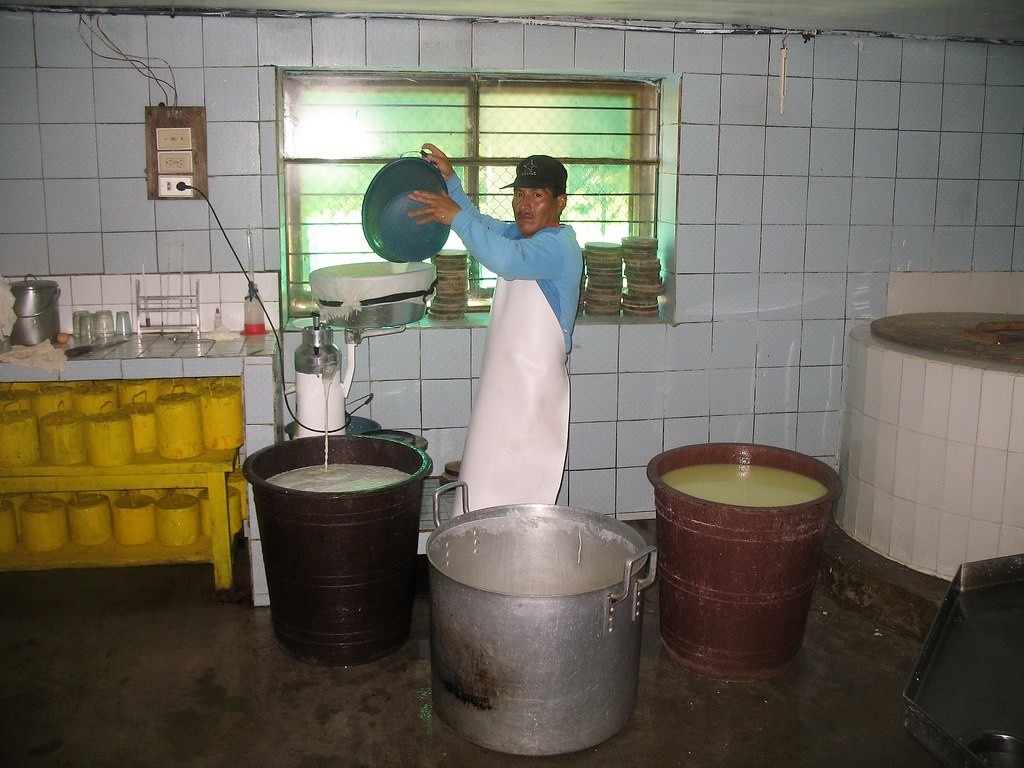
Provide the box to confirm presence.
[295,327,346,434]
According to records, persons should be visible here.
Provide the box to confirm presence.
[408,143,584,521]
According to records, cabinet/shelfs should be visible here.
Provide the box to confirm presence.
[0,448,240,590]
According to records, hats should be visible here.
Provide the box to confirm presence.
[499,155,568,188]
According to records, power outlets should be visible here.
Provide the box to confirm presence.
[156,175,193,197]
[157,152,193,174]
[156,128,191,150]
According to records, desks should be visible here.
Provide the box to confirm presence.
[0,330,281,606]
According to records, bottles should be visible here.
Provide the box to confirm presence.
[214,307,222,328]
[243,283,266,334]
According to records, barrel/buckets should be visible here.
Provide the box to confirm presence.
[240,434,435,668]
[5,272,65,347]
[424,499,656,756]
[644,441,842,685]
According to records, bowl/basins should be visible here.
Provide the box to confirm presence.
[360,155,452,263]
[309,261,436,328]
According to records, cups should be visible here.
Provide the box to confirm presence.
[95,310,115,338]
[116,311,131,337]
[73,311,94,341]
